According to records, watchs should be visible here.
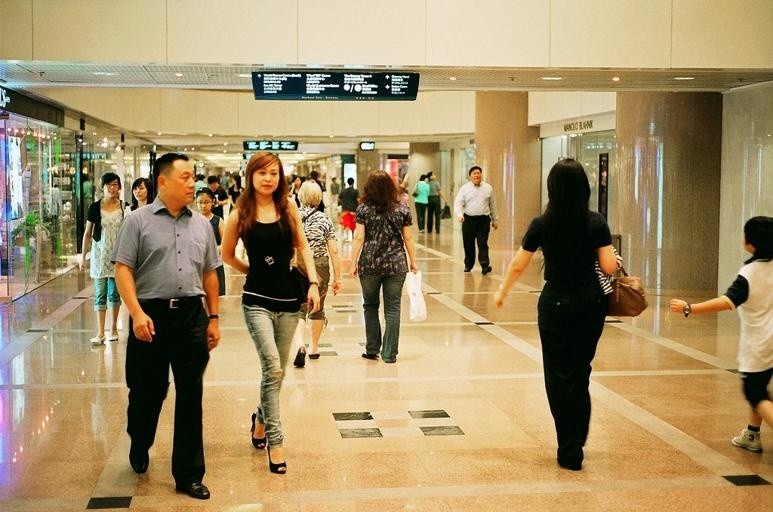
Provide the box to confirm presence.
[683,302,691,318]
[208,314,218,319]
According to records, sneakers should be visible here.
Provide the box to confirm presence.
[731,429,763,454]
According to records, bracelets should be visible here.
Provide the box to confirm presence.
[310,281,320,287]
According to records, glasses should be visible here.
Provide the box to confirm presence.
[105,183,119,186]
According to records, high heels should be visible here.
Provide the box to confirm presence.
[268,445,287,473]
[251,413,268,450]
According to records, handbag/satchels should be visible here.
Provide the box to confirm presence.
[441,203,451,219]
[302,208,319,222]
[406,270,428,322]
[603,262,650,317]
[374,204,411,271]
[290,247,310,303]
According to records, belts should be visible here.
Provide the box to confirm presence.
[137,296,201,307]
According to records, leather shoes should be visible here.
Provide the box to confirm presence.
[464,267,471,272]
[174,483,210,500]
[309,354,320,360]
[293,346,306,369]
[129,443,150,475]
[109,332,119,341]
[92,336,107,344]
[482,267,492,275]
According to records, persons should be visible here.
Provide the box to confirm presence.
[454,166,497,274]
[350,171,416,363]
[128,178,152,212]
[219,152,320,473]
[670,217,773,453]
[413,175,429,233]
[287,177,300,209]
[207,177,227,222]
[195,187,225,314]
[426,171,449,235]
[227,175,241,215]
[310,170,324,214]
[492,158,617,471]
[110,154,222,499]
[338,178,359,242]
[332,178,338,207]
[194,175,206,198]
[79,174,132,345]
[292,181,340,368]
